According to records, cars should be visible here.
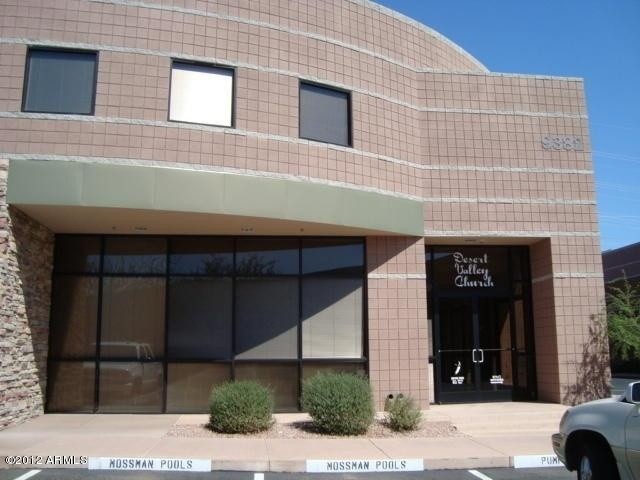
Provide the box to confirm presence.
[551,379,640,480]
